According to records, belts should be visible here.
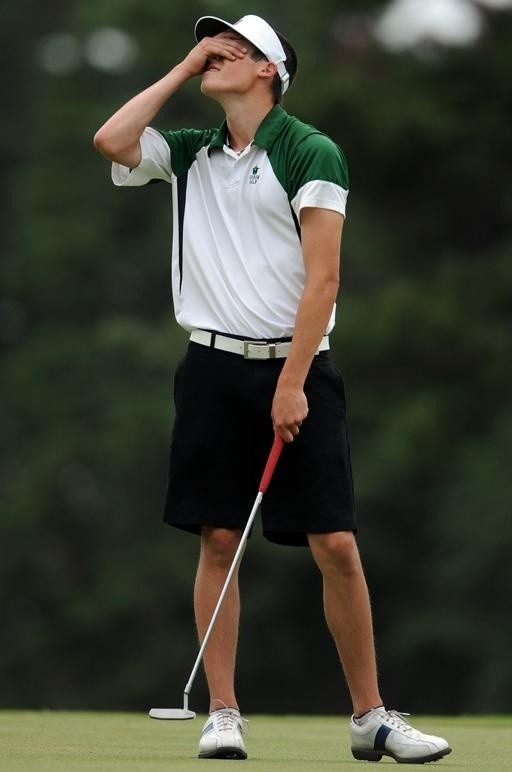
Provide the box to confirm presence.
[189,328,330,359]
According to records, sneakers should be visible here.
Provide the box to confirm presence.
[198,708,248,759]
[349,710,451,764]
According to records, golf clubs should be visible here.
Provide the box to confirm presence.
[149,435,284,721]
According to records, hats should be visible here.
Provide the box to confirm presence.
[194,14,290,94]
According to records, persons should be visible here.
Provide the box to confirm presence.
[93,14,453,764]
